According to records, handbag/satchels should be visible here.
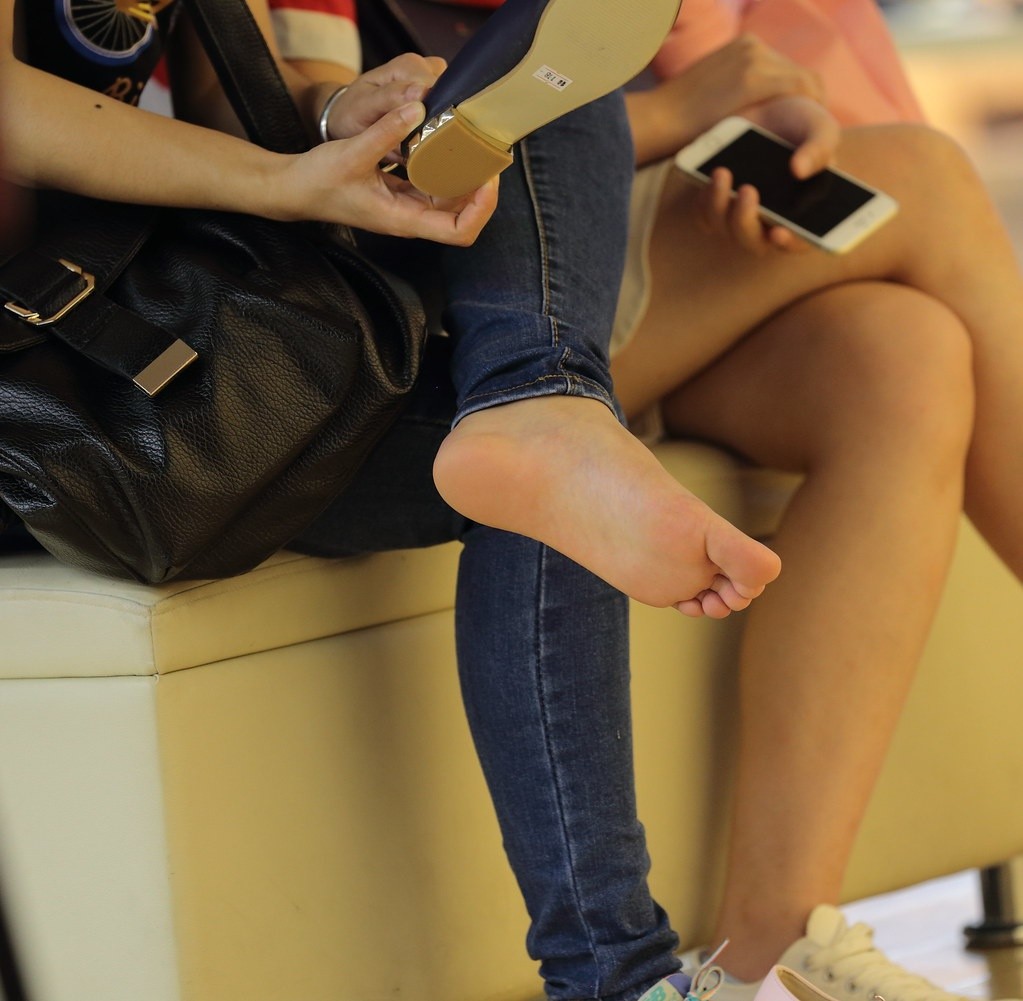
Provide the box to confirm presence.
[0,180,448,591]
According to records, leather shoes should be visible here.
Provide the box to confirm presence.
[406,0,682,198]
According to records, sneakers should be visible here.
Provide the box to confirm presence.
[694,912,981,1001]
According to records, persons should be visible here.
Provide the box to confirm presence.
[137,1,1022,1001]
[2,1,794,1001]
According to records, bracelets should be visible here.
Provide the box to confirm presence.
[318,85,402,173]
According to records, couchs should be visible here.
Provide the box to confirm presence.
[0,436,1023,1001]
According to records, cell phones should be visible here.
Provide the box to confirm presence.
[675,115,900,258]
